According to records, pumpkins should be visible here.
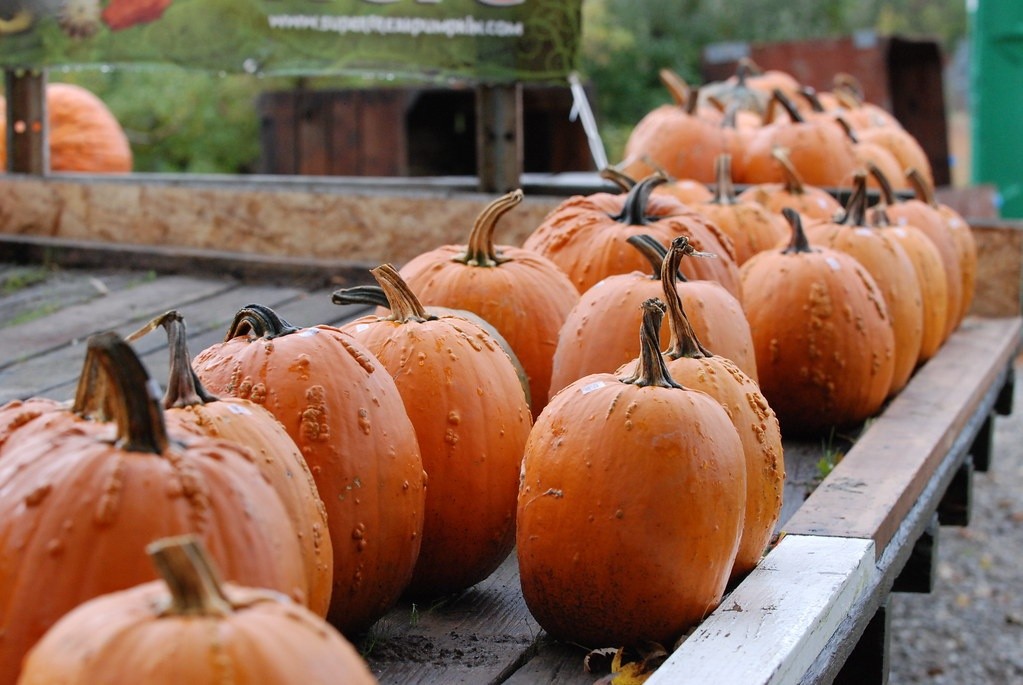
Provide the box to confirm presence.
[0,54,978,685]
